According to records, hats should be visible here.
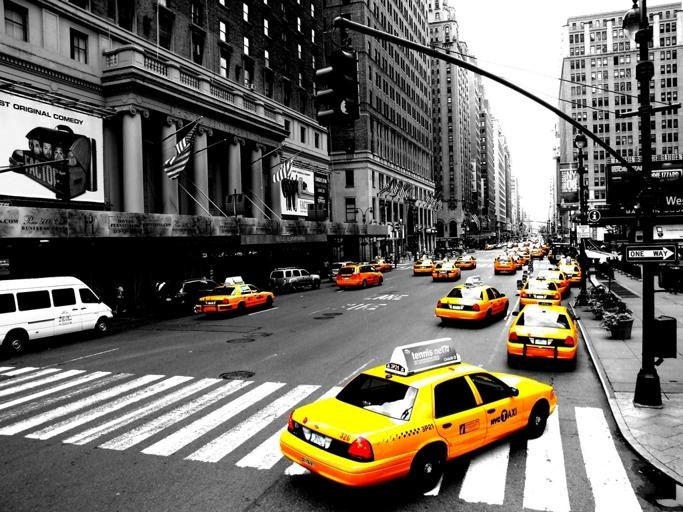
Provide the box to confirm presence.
[268,266,321,293]
[194,276,274,316]
[159,278,220,315]
[514,275,561,311]
[434,275,510,325]
[431,256,461,281]
[482,235,551,273]
[538,265,572,298]
[322,261,354,274]
[368,258,393,273]
[278,335,560,496]
[413,253,435,276]
[505,302,581,371]
[556,257,581,287]
[454,254,476,269]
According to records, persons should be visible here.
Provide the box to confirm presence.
[281,173,307,212]
[29,138,77,166]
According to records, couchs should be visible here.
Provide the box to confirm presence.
[315,58,334,129]
[334,47,361,122]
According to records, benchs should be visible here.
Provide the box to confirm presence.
[163,121,200,180]
[271,154,297,183]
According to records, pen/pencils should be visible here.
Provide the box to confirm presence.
[0,274,113,356]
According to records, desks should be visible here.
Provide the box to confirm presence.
[573,129,587,305]
[619,0,663,406]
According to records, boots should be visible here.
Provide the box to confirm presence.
[335,263,383,287]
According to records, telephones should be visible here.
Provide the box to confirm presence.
[625,242,679,265]
